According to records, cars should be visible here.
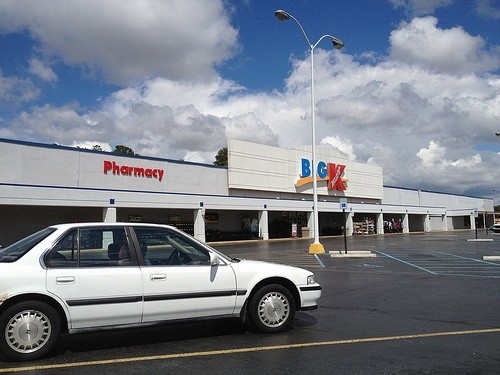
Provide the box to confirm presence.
[0,222,322,362]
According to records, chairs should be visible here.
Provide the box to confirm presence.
[45,250,68,267]
[108,243,131,266]
[139,240,153,266]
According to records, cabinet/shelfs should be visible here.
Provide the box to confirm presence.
[164,223,194,237]
[353,217,374,236]
[292,216,308,238]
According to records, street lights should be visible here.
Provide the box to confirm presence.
[274,8,346,256]
